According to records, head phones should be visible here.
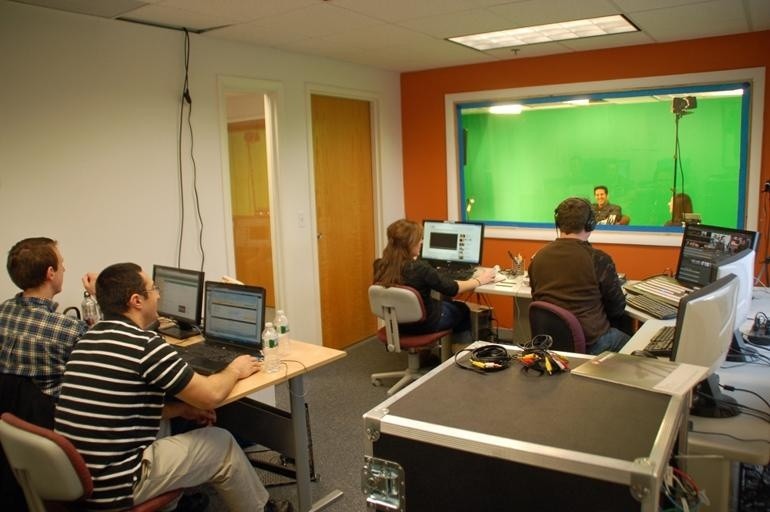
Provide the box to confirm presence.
[555,196,596,233]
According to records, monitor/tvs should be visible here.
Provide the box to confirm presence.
[670,273,741,418]
[153,265,205,340]
[710,248,755,363]
[419,220,484,270]
[674,223,762,288]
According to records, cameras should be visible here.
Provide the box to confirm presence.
[671,97,697,114]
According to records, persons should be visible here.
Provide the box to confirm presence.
[591,186,622,224]
[527,197,633,356]
[0,237,97,401]
[52,261,294,512]
[664,192,699,226]
[372,218,497,369]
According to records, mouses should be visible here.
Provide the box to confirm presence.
[631,350,658,359]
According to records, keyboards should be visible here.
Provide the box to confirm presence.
[436,269,473,281]
[643,326,675,357]
[625,294,679,320]
[623,275,703,308]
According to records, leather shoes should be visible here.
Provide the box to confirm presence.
[264,498,294,512]
[173,492,209,512]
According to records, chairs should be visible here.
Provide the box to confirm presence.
[529,301,587,354]
[368,283,453,396]
[0,412,183,512]
[0,372,52,427]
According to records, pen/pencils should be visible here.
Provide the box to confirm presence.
[508,250,523,269]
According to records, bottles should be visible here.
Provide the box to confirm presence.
[82,291,97,324]
[262,322,281,373]
[272,309,290,357]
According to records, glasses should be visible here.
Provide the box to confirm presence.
[145,285,160,294]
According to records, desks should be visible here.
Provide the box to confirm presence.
[160,316,349,512]
[457,266,770,511]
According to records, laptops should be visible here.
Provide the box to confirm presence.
[176,281,266,376]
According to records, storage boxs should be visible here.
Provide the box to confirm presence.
[360,339,692,512]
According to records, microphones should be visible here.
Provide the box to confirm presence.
[466,199,476,214]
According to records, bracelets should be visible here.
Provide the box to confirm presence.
[475,277,482,285]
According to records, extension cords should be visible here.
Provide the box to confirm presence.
[748,317,770,345]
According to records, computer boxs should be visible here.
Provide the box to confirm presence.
[456,300,493,342]
